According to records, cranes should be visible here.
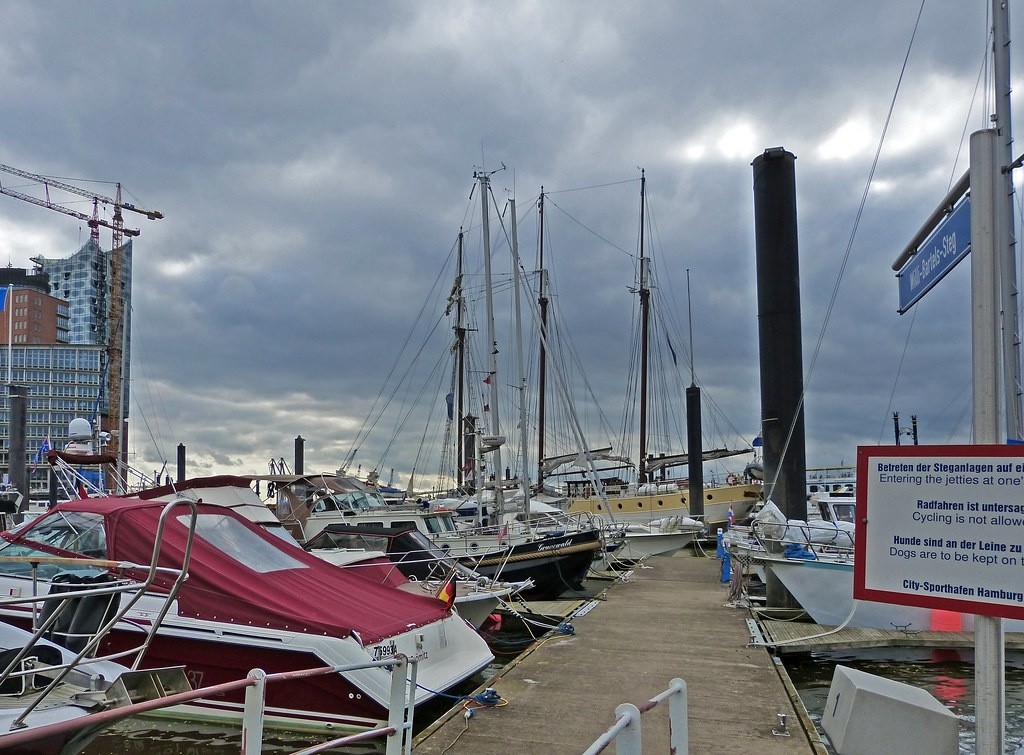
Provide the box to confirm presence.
[0,164,164,496]
[0,187,140,247]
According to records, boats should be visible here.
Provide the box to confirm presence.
[750,519,1024,634]
[722,476,856,571]
[592,517,704,571]
[300,524,481,581]
[0,499,197,755]
[295,509,602,602]
[0,499,496,744]
[305,548,536,632]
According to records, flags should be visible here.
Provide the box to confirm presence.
[437,573,457,611]
[34,435,51,465]
[727,502,736,527]
[497,523,508,544]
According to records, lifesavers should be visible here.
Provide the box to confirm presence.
[726,475,737,486]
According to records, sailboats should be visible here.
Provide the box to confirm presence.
[241,139,568,523]
[561,167,763,550]
